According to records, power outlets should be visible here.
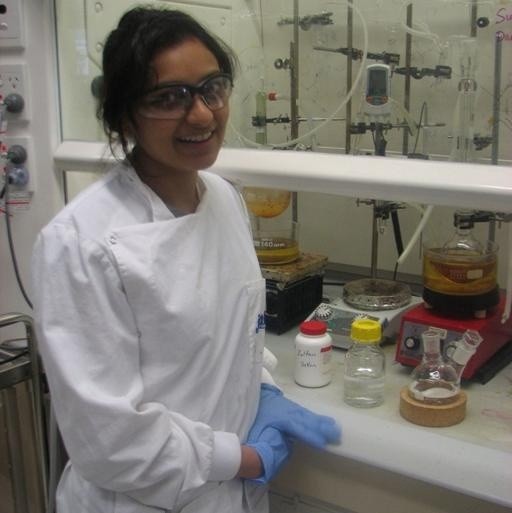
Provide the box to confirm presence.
[0,63,36,195]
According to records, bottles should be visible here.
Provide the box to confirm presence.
[293,321,332,388]
[344,319,385,407]
[409,328,483,406]
[437,215,488,283]
[239,92,291,217]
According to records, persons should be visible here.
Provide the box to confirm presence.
[28,5,342,513]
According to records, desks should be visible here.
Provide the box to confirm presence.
[249,278,511,512]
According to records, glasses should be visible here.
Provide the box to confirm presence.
[135,74,233,120]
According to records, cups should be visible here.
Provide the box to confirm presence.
[250,219,299,266]
[423,235,499,295]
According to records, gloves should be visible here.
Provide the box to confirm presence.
[241,383,343,486]
